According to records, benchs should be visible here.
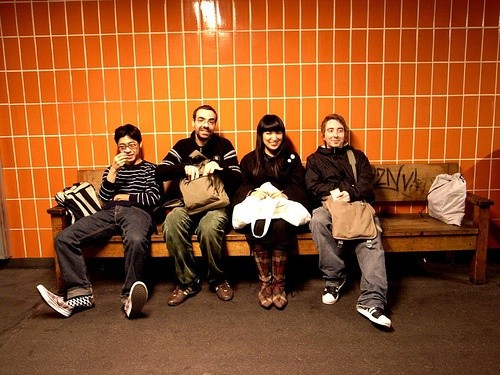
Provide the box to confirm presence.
[47,163,495,292]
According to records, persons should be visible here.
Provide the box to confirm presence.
[233,114,306,309]
[306,114,391,328]
[155,105,241,306]
[35,123,161,318]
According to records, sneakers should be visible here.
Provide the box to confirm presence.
[356,305,391,328]
[124,281,148,318]
[322,277,345,304]
[215,281,234,300]
[36,284,73,317]
[167,278,201,306]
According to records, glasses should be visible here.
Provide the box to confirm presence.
[118,142,139,150]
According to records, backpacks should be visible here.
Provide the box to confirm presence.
[427,173,467,226]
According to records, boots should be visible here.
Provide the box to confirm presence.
[271,250,287,310]
[254,250,273,310]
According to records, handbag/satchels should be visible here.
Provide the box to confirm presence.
[180,150,230,216]
[232,181,311,238]
[54,181,102,225]
[321,195,378,240]
